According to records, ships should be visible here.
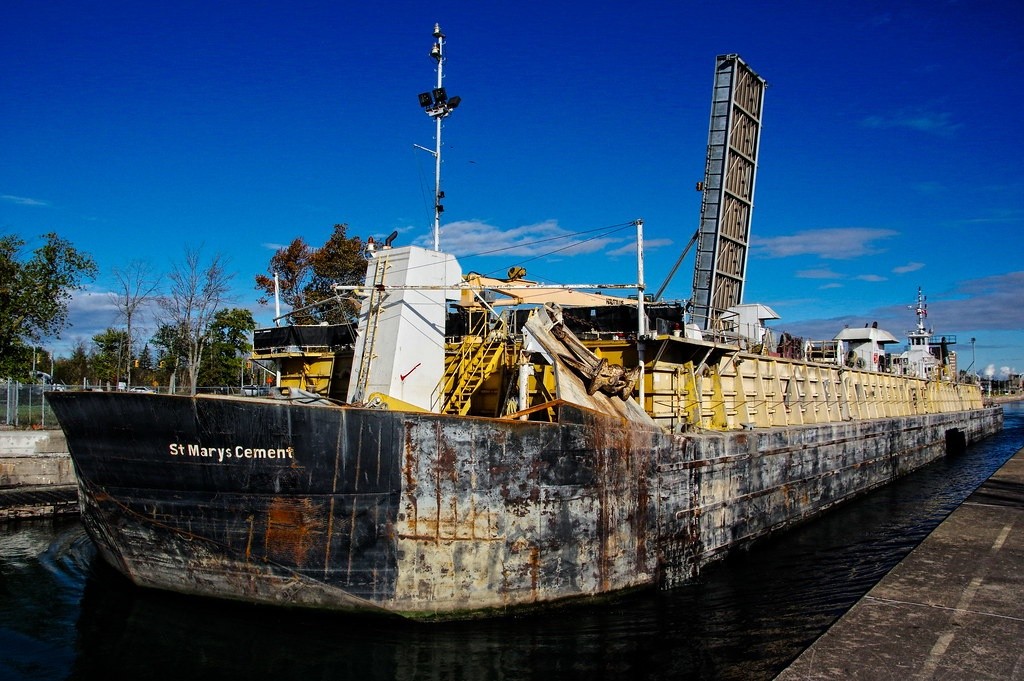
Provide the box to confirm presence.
[43,21,1004,620]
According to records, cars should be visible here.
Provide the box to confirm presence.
[240,385,263,396]
[126,386,155,394]
[3,372,64,396]
